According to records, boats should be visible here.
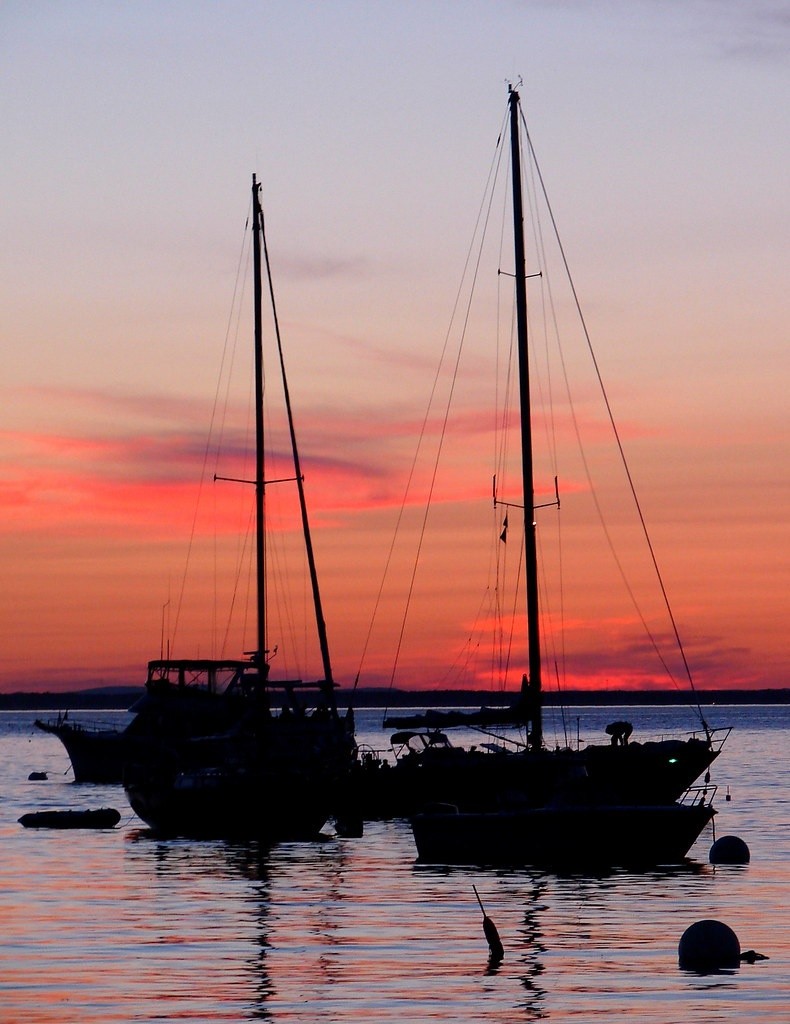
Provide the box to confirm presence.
[380,700,529,723]
[124,777,349,844]
[405,804,717,871]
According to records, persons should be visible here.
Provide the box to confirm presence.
[605,721,632,745]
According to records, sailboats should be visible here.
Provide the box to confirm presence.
[348,82,733,799]
[32,169,356,781]
